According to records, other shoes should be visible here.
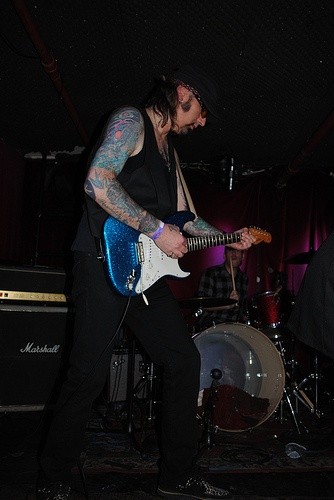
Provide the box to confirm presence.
[37,473,72,500]
[157,471,231,500]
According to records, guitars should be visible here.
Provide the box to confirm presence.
[100,207,273,296]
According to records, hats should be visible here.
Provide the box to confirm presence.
[172,58,225,120]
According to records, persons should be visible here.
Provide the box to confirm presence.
[36,71,256,500]
[288,230,334,360]
[197,246,250,329]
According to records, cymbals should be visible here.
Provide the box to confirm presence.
[284,251,317,265]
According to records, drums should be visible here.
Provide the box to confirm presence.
[249,290,293,330]
[188,322,287,431]
[254,270,282,292]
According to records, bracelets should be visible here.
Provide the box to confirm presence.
[151,221,165,240]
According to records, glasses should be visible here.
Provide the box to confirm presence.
[194,96,210,119]
